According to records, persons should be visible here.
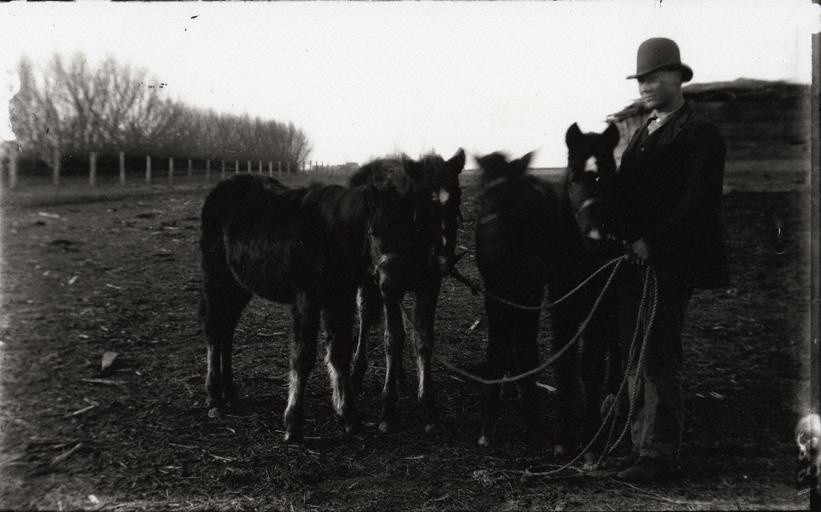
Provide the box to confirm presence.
[612,36,733,485]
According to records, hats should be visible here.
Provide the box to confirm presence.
[626,37,692,83]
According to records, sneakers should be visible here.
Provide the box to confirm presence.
[615,451,667,481]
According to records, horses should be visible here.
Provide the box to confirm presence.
[474,152,562,449]
[549,121,629,457]
[197,172,414,444]
[347,148,466,438]
